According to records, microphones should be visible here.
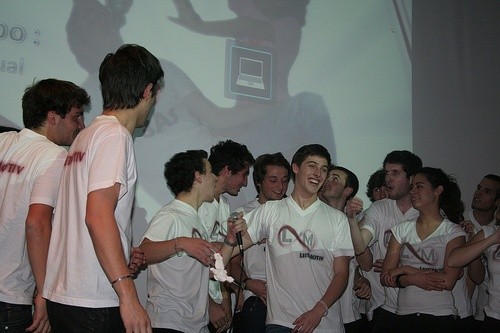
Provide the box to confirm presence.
[231,212,244,258]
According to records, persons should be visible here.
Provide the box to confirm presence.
[141,138,500,333]
[0,78,91,333]
[41,43,165,333]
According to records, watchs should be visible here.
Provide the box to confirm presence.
[240,277,252,291]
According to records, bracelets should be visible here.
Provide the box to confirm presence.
[317,299,328,317]
[396,274,405,288]
[112,274,131,284]
[175,238,183,257]
